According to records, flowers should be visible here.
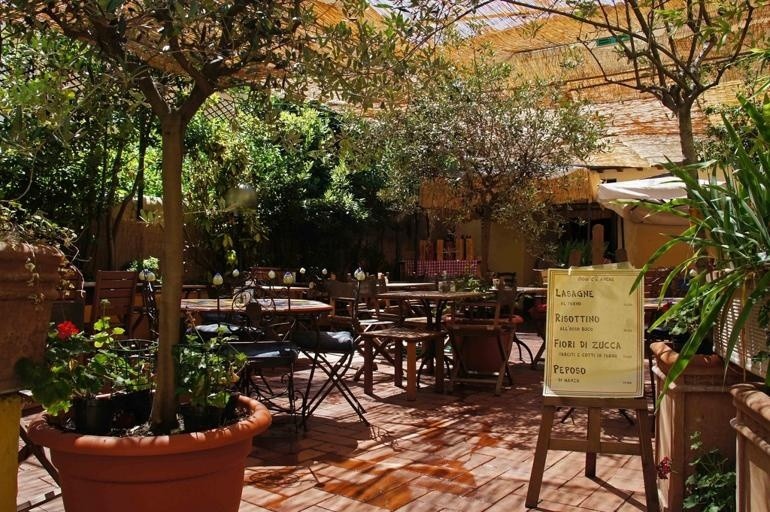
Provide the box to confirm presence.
[20,296,248,435]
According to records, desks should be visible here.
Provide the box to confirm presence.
[77,264,679,512]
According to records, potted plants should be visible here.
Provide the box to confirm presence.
[0,199,83,394]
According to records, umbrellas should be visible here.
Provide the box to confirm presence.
[592,172,728,227]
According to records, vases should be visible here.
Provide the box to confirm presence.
[28,389,273,512]
